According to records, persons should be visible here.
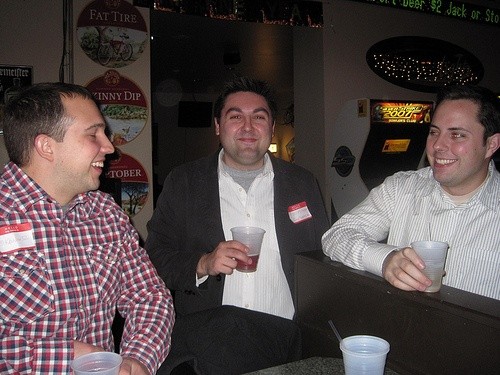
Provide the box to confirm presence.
[320,84,500,301]
[0,82,176,375]
[143,76,331,375]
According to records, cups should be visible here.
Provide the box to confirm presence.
[70,351,123,375]
[338,336,390,374]
[410,240,448,293]
[230,226,266,273]
